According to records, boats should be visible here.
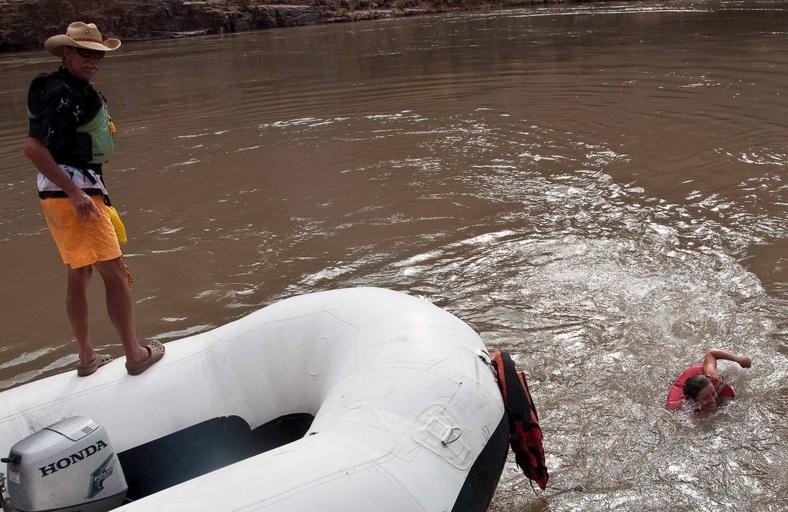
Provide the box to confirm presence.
[0,279,509,512]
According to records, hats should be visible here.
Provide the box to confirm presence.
[45,22,121,56]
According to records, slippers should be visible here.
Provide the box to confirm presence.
[77,354,113,376]
[125,341,164,374]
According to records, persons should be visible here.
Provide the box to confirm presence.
[680,347,753,415]
[23,22,166,375]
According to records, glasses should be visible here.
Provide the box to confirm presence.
[76,48,106,59]
